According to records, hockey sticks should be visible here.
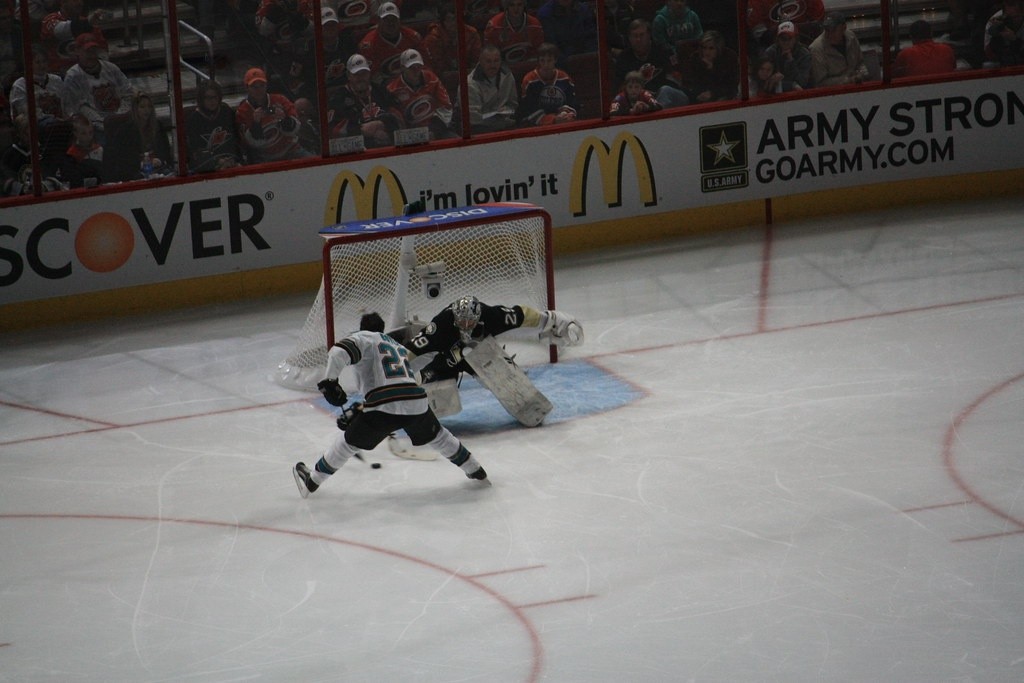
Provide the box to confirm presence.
[338,403,383,471]
[389,431,438,462]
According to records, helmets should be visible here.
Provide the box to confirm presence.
[452,295,481,331]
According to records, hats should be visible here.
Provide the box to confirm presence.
[376,1,400,20]
[320,7,339,26]
[776,20,799,39]
[244,68,267,89]
[400,48,424,68]
[819,12,846,29]
[75,32,100,50]
[345,52,370,75]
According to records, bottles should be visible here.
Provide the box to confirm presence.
[775,80,783,93]
[143,152,152,178]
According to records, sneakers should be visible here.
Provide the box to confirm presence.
[292,461,319,500]
[466,466,492,487]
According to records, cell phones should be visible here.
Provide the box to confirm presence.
[263,106,277,114]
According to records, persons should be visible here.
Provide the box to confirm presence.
[293,295,585,499]
[0,0,1024,197]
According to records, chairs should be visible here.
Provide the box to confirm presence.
[0,0,881,187]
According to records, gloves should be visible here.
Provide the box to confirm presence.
[538,309,585,349]
[317,379,347,407]
[336,401,364,431]
